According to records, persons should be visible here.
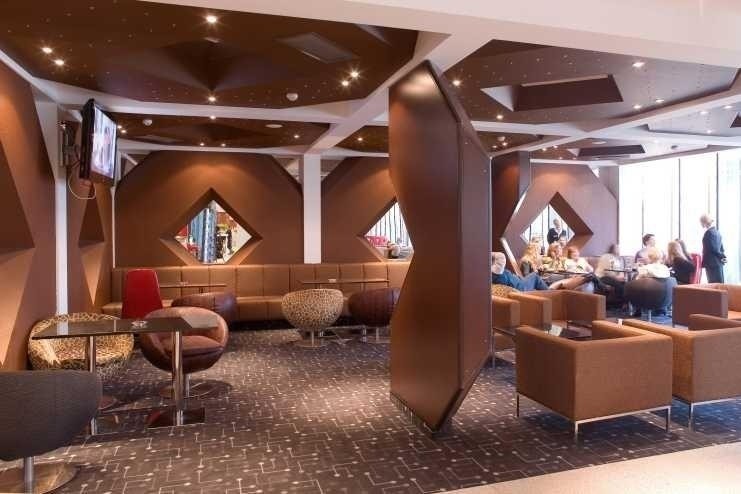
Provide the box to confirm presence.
[519,233,696,317]
[385,237,404,258]
[548,217,567,244]
[701,215,728,283]
[492,249,550,292]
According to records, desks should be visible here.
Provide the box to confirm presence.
[489,317,594,370]
[31,318,219,444]
[160,282,228,296]
[298,277,388,344]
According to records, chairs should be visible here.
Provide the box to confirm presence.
[0,372,103,493]
[670,282,741,328]
[282,290,345,349]
[174,292,237,327]
[621,312,741,421]
[488,256,705,323]
[138,308,231,429]
[351,289,400,343]
[488,294,529,353]
[507,288,607,329]
[511,318,677,444]
[121,267,163,320]
[28,312,135,437]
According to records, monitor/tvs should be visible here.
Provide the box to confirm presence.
[78,98,117,186]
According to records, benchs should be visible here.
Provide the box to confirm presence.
[101,262,410,327]
